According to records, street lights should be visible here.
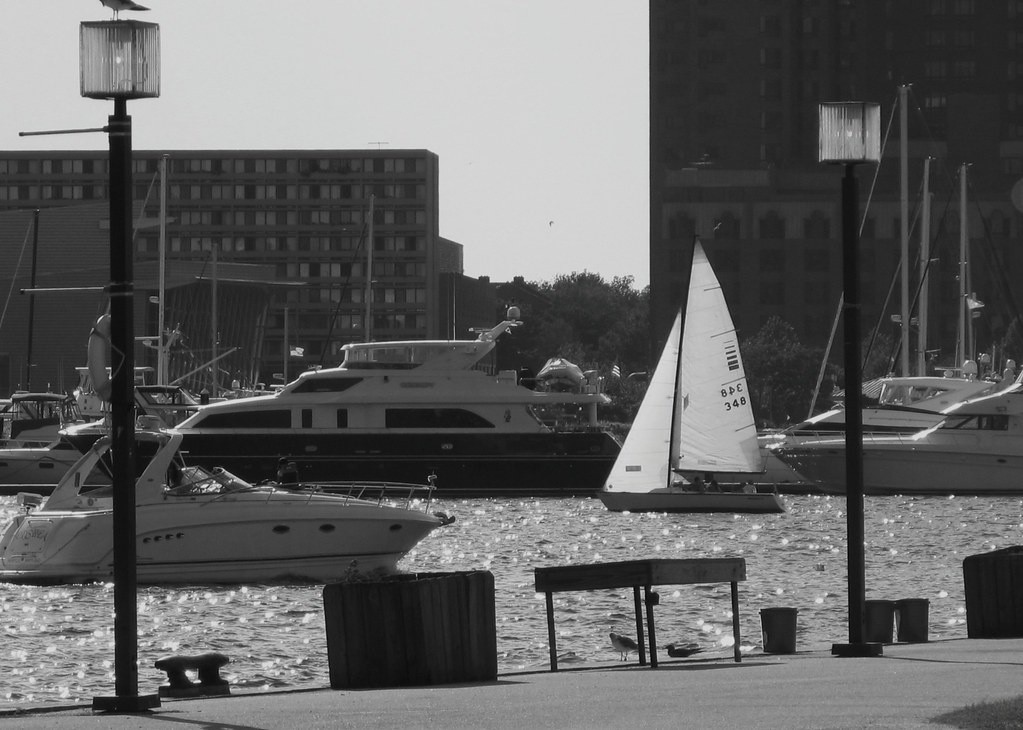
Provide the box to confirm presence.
[76,21,164,716]
[815,101,885,658]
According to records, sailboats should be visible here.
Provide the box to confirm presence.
[592,234,789,515]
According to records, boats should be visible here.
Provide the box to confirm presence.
[1,422,456,588]
[1,199,643,499]
[762,83,1022,499]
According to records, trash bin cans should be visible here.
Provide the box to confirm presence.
[893,598,930,643]
[758,607,798,653]
[865,599,894,643]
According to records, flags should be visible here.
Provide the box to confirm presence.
[290,345,304,357]
